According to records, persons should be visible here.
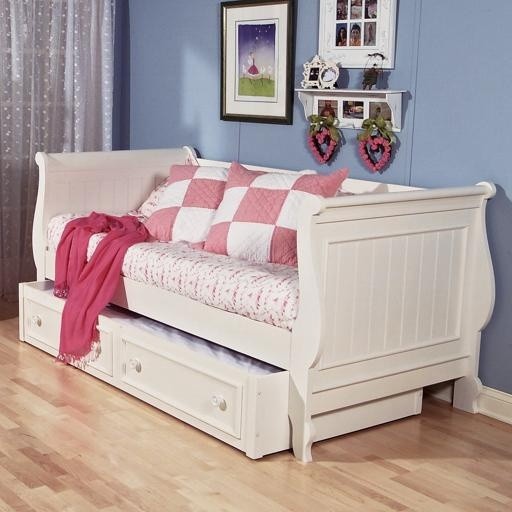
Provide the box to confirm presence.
[320,100,335,118]
[337,27,347,45]
[350,22,361,45]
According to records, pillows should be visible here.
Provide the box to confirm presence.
[132,162,349,267]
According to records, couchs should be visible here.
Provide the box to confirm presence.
[18,145,499,463]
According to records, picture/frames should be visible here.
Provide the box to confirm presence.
[220,0,294,125]
[301,55,326,89]
[318,61,340,90]
[317,0,397,69]
